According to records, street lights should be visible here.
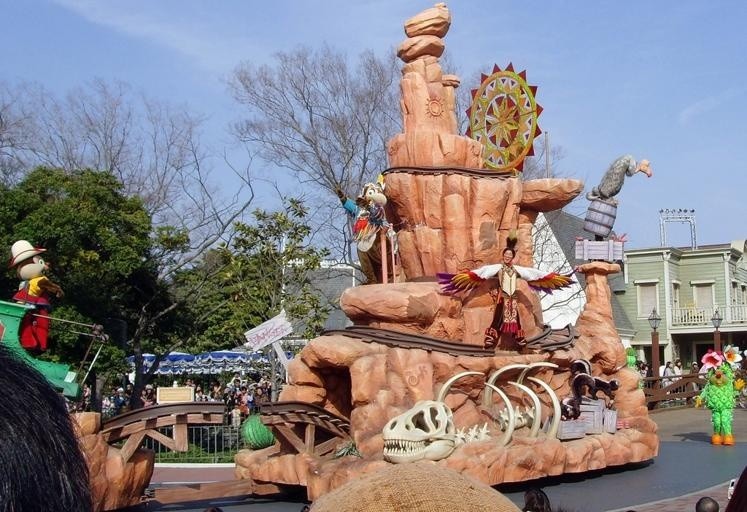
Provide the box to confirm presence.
[712,307,722,352]
[648,306,662,378]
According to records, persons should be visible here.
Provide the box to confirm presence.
[695,497,720,512]
[636,358,653,377]
[658,359,700,403]
[0,342,96,512]
[63,383,157,422]
[184,372,273,429]
[523,489,552,512]
[434,231,578,352]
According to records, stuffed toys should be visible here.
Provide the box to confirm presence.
[693,343,746,446]
[8,239,64,351]
[332,180,399,285]
